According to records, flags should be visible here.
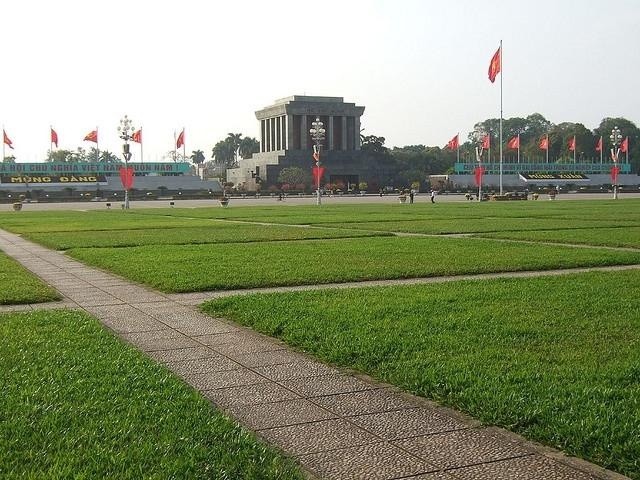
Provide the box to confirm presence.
[487,47,500,83]
[619,139,627,153]
[482,134,489,149]
[51,129,58,147]
[4,132,14,149]
[507,136,519,149]
[177,131,184,149]
[596,138,602,152]
[83,131,97,142]
[568,137,574,151]
[448,136,458,150]
[539,137,547,149]
[130,129,141,143]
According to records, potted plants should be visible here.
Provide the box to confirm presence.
[410,181,420,195]
[241,181,369,198]
[433,182,497,195]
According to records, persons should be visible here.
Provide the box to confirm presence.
[410,191,416,204]
[430,190,434,203]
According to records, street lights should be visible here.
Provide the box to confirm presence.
[310,118,326,205]
[610,126,623,199]
[468,124,488,201]
[117,115,136,208]
